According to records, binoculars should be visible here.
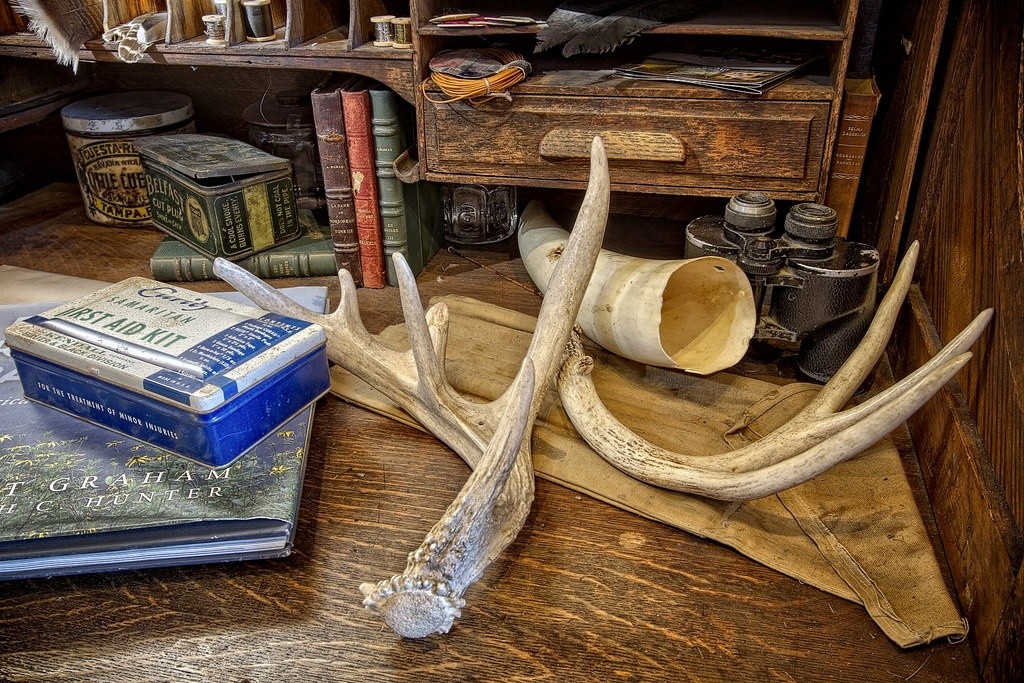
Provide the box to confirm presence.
[682,191,879,395]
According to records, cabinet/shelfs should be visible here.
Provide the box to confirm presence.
[410,0,859,203]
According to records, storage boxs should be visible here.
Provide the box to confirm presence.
[5,275,331,470]
[134,134,303,262]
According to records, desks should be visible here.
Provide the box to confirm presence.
[0,178,982,682]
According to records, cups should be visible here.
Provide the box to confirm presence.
[58,89,196,229]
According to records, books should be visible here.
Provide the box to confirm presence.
[825,75,880,237]
[311,72,443,289]
[0,285,327,580]
[611,47,818,95]
[151,208,337,284]
